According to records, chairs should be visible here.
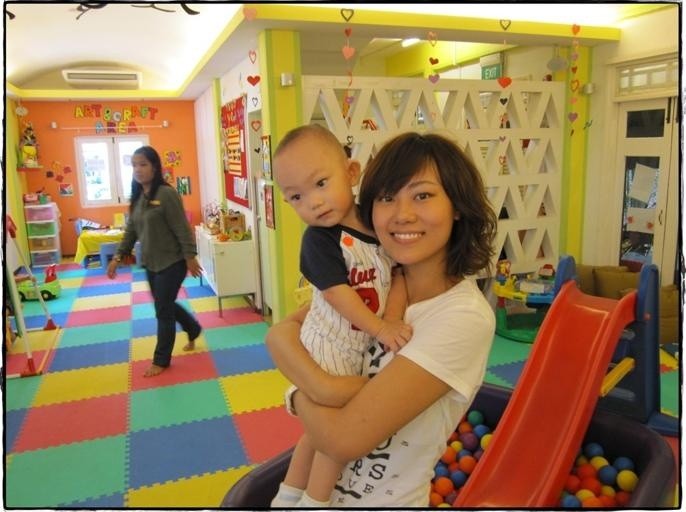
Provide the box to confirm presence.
[74,218,144,270]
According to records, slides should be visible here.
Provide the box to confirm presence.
[452,279,637,508]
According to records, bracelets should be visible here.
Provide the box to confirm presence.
[285,385,299,417]
[111,255,120,262]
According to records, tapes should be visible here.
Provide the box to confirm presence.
[230,226,243,241]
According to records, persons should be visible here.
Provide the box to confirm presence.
[271,125,412,508]
[107,146,203,377]
[266,132,497,508]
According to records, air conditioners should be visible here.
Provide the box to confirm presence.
[61,68,143,88]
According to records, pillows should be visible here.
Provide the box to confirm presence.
[576,264,681,345]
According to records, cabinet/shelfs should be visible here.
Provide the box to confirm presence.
[194,225,259,318]
[23,201,61,268]
